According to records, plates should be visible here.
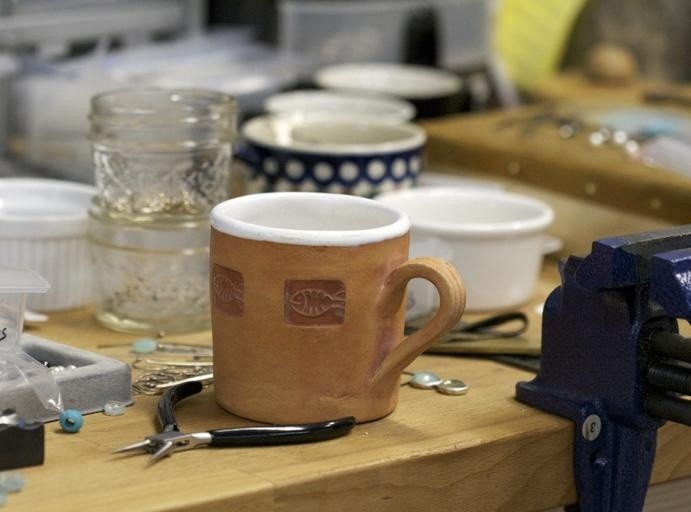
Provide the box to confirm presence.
[0,331,134,426]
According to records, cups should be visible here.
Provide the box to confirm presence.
[260,92,414,142]
[207,190,467,425]
[311,62,471,120]
[90,87,238,336]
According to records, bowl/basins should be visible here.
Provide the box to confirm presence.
[373,185,555,313]
[242,117,426,200]
[1,174,98,311]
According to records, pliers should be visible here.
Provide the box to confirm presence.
[110,379,357,467]
